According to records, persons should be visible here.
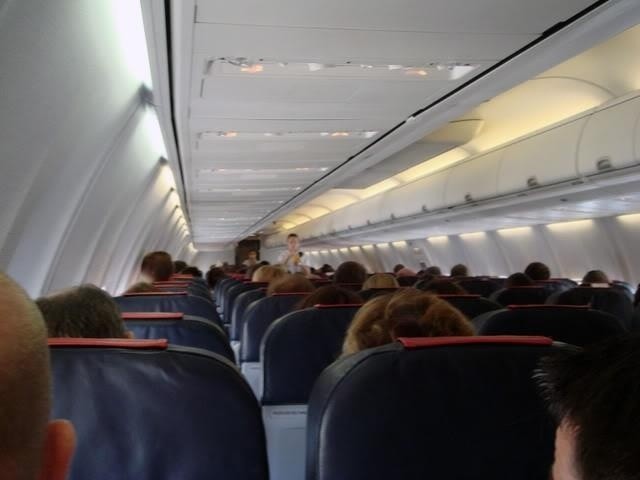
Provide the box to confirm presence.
[206,235,640,355]
[140,251,202,285]
[33,284,135,338]
[553,369,640,479]
[0,273,77,480]
